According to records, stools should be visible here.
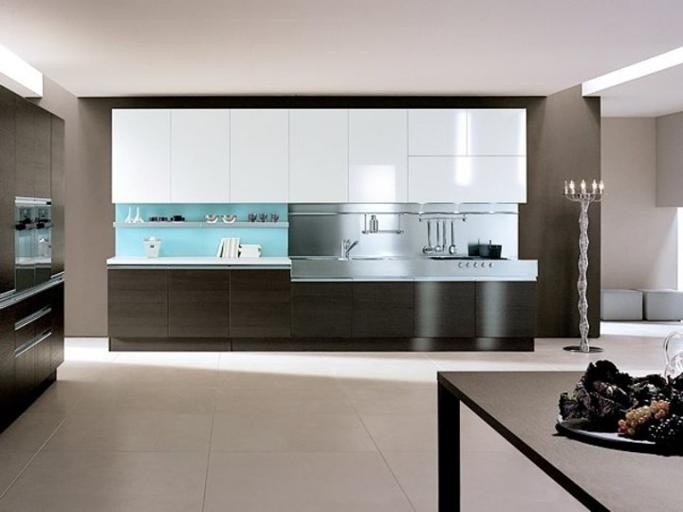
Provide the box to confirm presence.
[639,289,683,322]
[599,287,643,321]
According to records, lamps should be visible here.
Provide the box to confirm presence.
[560,177,607,353]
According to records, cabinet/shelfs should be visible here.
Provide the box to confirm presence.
[108,108,407,202]
[291,281,540,353]
[409,107,530,204]
[0,86,65,410]
[105,265,291,352]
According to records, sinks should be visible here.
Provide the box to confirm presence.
[290,256,338,262]
[352,257,387,261]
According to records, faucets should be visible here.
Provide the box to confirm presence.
[341,240,359,257]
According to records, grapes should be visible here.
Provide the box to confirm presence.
[618,399,683,446]
